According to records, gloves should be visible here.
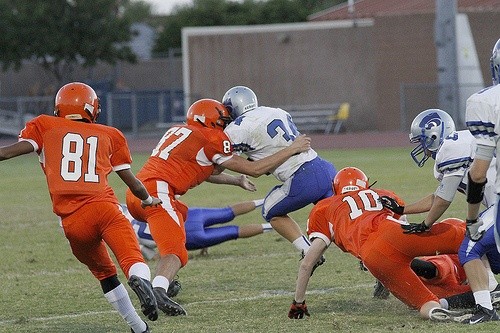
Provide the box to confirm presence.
[401,220,431,234]
[288,299,310,319]
[377,195,404,215]
[465,218,486,242]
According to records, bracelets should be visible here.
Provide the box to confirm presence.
[141,196,152,204]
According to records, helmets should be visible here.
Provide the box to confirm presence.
[222,85,257,118]
[490,37,500,84]
[186,98,229,130]
[53,81,99,123]
[334,167,369,196]
[409,108,456,168]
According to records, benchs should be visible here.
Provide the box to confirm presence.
[282,102,350,135]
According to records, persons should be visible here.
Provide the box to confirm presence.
[120,199,274,263]
[288,167,500,323]
[211,86,390,299]
[380,109,500,324]
[466,38,500,254]
[0,82,163,333]
[126,98,312,316]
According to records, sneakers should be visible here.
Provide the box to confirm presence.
[461,304,499,325]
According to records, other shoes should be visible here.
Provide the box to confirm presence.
[359,260,368,272]
[295,248,325,282]
[154,287,187,316]
[490,289,500,308]
[428,307,473,323]
[373,280,390,299]
[130,322,150,333]
[167,281,181,298]
[128,274,158,321]
